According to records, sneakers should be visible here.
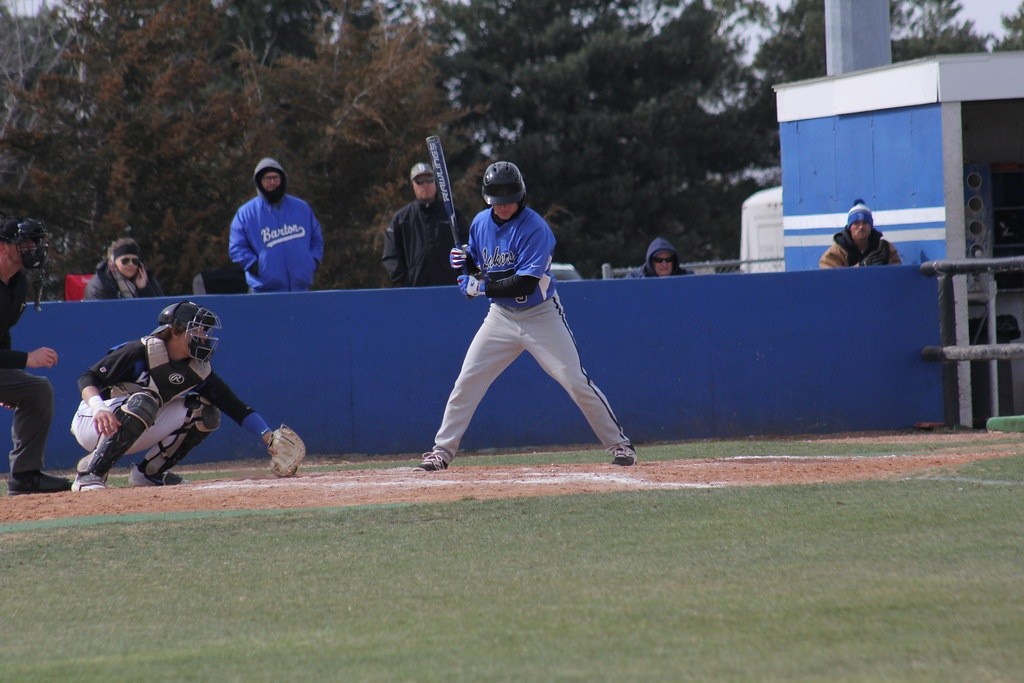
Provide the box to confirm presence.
[71,472,108,493]
[604,443,638,466]
[7,470,70,494]
[421,450,448,471]
[128,462,182,487]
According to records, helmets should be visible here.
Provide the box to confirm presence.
[482,162,526,209]
[149,300,215,335]
[0,214,45,243]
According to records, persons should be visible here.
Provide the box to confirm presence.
[819,198,903,268]
[382,162,472,287]
[624,238,695,278]
[413,161,638,470]
[227,156,324,293]
[68,300,276,491]
[0,215,72,495]
[83,236,164,299]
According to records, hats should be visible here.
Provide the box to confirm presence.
[846,198,874,229]
[410,163,434,180]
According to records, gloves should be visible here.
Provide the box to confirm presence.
[457,274,486,297]
[246,260,259,276]
[449,244,467,269]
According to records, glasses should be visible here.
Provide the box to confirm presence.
[653,256,674,264]
[414,177,435,186]
[262,175,282,182]
[119,257,140,267]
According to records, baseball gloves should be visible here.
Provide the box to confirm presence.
[267,422,306,478]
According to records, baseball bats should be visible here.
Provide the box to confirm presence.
[426,135,473,300]
